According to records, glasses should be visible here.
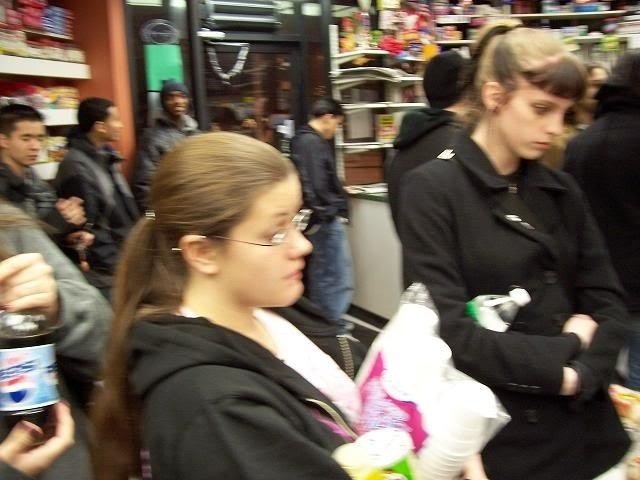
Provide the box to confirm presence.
[206,208,313,247]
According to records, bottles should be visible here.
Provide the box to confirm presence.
[462,286,531,334]
[0,310,65,448]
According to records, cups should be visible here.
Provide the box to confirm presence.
[328,302,500,480]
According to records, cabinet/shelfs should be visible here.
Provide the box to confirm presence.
[0,0,127,182]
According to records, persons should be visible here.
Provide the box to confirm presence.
[0,16,640,479]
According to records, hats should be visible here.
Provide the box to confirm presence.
[423,50,474,108]
[160,78,189,109]
